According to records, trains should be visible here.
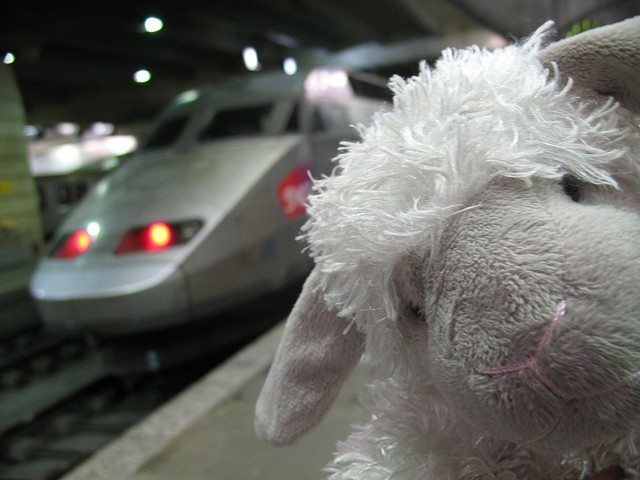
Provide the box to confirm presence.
[29,49,394,334]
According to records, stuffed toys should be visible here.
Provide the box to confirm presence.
[254,16,640,480]
